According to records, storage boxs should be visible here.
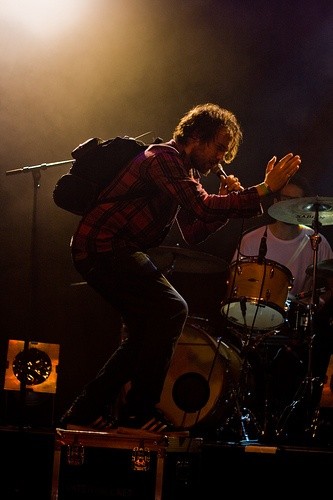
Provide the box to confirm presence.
[50,428,203,500]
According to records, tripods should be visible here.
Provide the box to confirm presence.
[259,211,333,448]
[211,328,269,444]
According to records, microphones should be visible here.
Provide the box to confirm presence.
[212,164,242,193]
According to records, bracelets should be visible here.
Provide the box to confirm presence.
[260,183,272,197]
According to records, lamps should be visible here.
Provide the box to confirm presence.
[3,339,60,407]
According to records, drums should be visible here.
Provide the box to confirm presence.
[218,255,295,331]
[122,321,251,435]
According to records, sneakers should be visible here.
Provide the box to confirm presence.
[117,410,170,437]
[60,394,119,435]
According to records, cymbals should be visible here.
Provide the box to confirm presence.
[268,196,333,225]
[153,244,230,275]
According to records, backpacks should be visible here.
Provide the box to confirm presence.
[52,137,187,217]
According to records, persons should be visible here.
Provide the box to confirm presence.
[59,104,301,438]
[233,177,333,444]
